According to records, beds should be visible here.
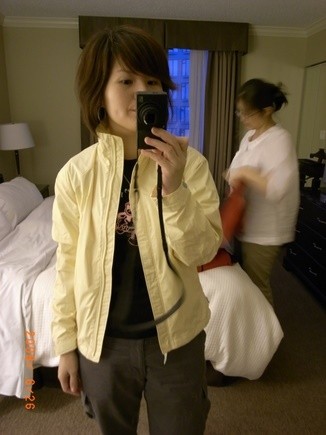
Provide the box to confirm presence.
[0,195,284,399]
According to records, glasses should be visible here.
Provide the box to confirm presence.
[235,107,266,121]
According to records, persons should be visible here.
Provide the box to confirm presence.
[226,77,299,308]
[51,25,221,435]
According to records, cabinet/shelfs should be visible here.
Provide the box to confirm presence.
[281,158,326,312]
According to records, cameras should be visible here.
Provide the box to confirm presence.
[137,91,169,149]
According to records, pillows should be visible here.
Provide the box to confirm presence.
[0,176,43,239]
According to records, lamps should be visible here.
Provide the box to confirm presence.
[0,122,35,176]
